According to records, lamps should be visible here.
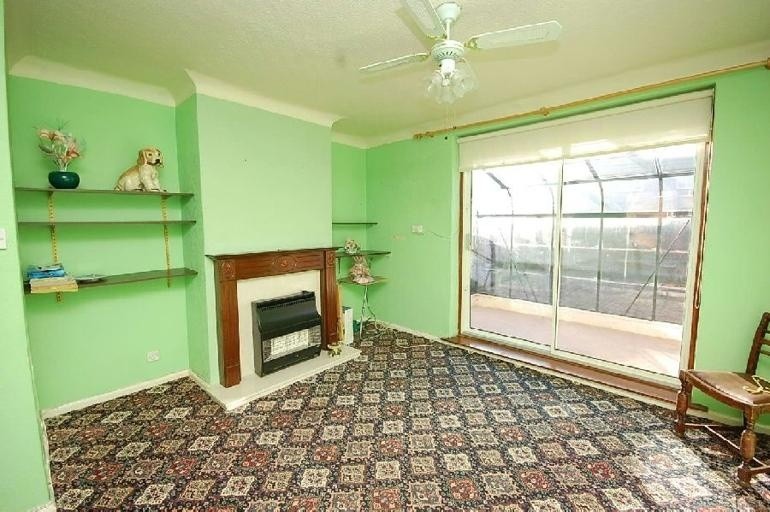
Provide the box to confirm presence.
[427,59,473,103]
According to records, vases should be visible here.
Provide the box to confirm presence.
[49,171,79,189]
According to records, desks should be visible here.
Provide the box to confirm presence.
[338,276,389,340]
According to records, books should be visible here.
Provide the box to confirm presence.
[27,263,78,294]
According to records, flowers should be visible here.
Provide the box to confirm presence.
[38,129,85,171]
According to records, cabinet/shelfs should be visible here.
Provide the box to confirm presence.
[332,223,390,258]
[14,187,197,295]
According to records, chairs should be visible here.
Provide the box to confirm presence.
[675,313,770,486]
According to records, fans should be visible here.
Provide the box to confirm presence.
[359,0,562,73]
